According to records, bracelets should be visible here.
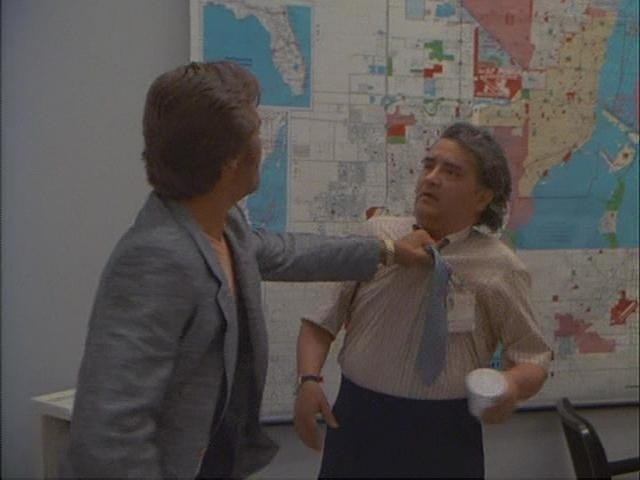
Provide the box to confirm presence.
[384,240,396,267]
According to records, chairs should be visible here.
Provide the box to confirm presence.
[557,397,639,480]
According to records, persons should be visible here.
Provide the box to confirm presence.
[293,121,554,479]
[58,60,436,480]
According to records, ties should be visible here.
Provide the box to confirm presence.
[420,244,448,387]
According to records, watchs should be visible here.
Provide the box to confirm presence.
[297,375,323,385]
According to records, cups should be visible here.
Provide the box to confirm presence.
[462,366,510,418]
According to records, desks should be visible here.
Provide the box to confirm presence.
[29,389,75,480]
